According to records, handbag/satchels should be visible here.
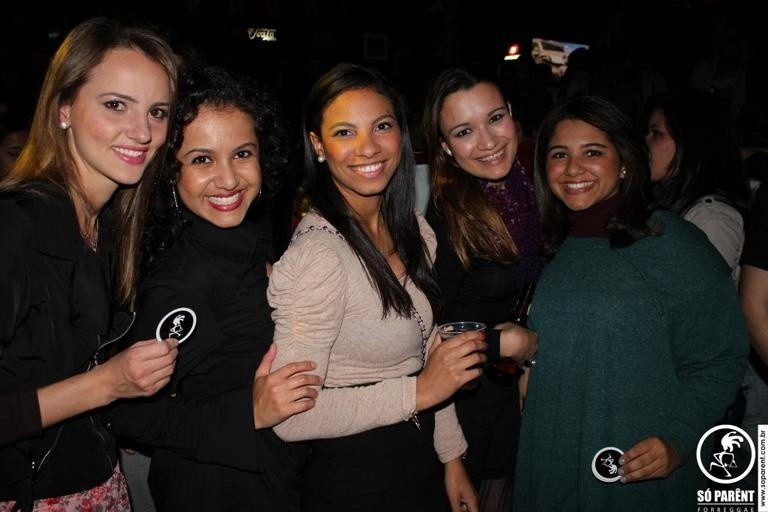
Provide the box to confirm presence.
[440,258,537,377]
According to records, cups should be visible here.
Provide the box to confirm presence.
[438,321,486,391]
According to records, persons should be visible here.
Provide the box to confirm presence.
[1,16,768,512]
